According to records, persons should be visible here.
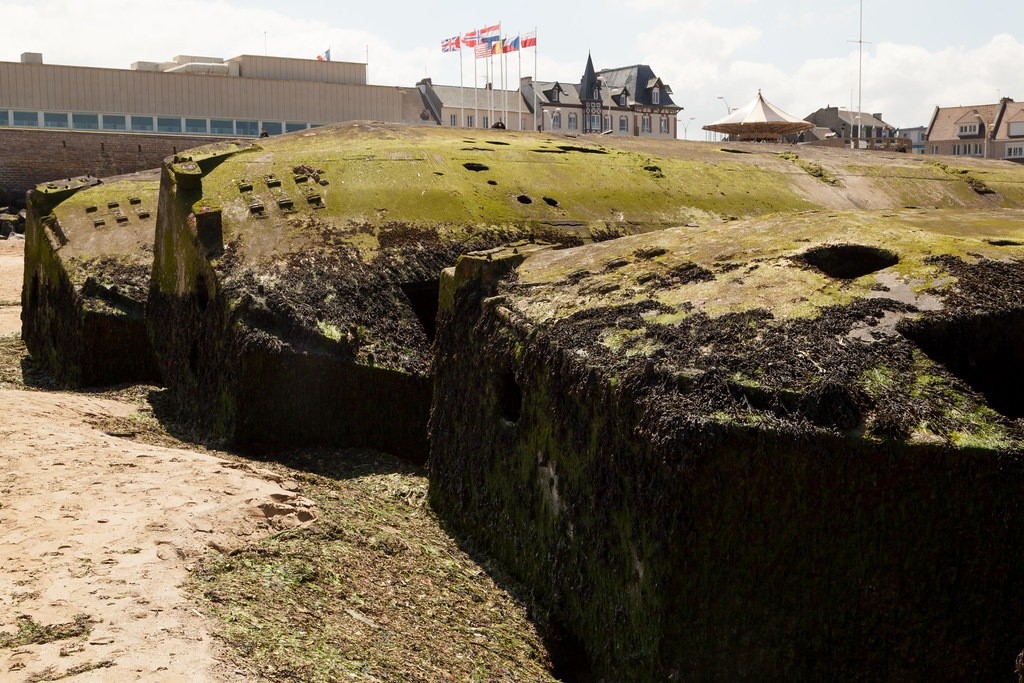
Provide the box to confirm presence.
[259,128,269,139]
[899,143,908,152]
[895,144,901,152]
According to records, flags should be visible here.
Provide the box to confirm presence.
[492,38,508,56]
[475,41,493,57]
[507,36,521,53]
[441,35,460,53]
[480,25,502,44]
[461,30,480,46]
[316,49,330,61]
[521,31,536,48]
